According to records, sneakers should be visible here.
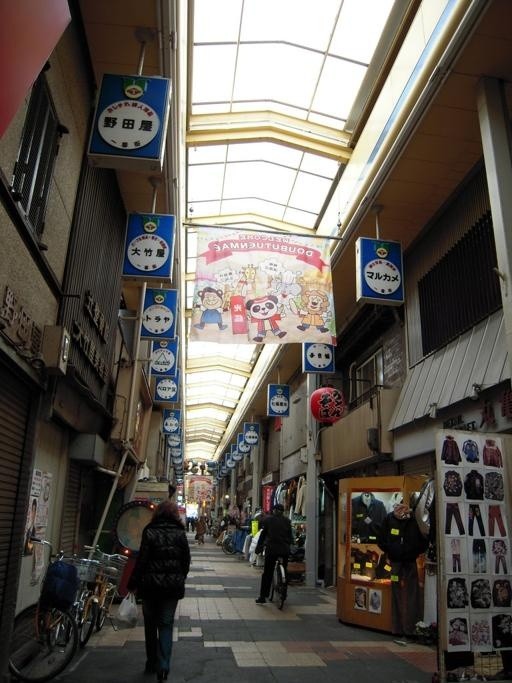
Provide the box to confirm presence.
[146,665,168,681]
[256,598,266,603]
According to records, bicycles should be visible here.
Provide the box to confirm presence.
[258,548,289,611]
[7,535,130,681]
[220,534,237,555]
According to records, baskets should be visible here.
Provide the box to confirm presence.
[75,553,128,583]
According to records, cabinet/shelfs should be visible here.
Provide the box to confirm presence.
[336,474,431,634]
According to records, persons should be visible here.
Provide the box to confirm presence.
[22,498,41,554]
[376,503,430,635]
[187,515,211,545]
[127,499,191,682]
[248,503,294,604]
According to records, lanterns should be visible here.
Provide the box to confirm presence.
[309,385,346,424]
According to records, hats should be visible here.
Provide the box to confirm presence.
[272,503,285,511]
